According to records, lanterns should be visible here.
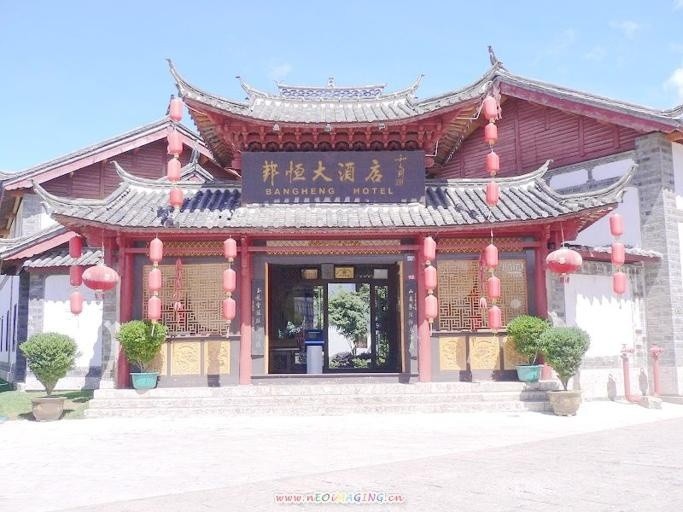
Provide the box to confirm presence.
[484,243,502,332]
[423,236,438,324]
[610,214,627,294]
[148,238,163,325]
[69,236,83,317]
[482,96,500,207]
[82,262,120,295]
[545,247,583,284]
[222,238,237,323]
[167,98,184,208]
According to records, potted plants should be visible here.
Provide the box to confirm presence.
[505,312,549,384]
[541,326,592,419]
[115,318,168,389]
[18,330,77,422]
